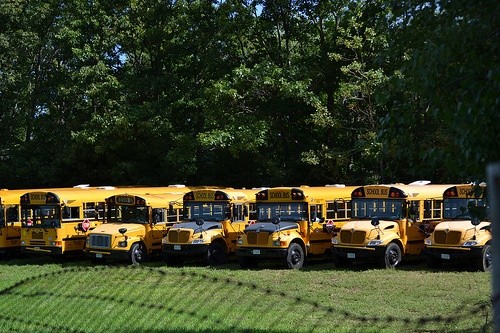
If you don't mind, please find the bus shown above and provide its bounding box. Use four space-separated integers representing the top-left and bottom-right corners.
0 189 42 248
235 185 368 270
423 184 493 273
18 187 193 255
87 194 191 266
332 184 471 269
160 188 264 266
